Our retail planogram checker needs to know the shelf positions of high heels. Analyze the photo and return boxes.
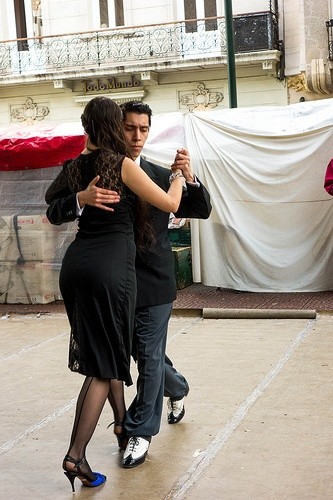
[113,421,126,448]
[63,454,106,492]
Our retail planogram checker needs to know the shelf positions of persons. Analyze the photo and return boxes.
[45,101,212,467]
[44,97,187,494]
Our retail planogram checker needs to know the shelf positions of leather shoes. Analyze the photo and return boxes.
[167,385,189,424]
[121,436,151,468]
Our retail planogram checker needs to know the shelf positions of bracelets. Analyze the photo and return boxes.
[169,174,184,183]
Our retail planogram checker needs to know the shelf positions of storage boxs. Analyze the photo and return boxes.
[167,218,193,290]
[0,217,78,304]
